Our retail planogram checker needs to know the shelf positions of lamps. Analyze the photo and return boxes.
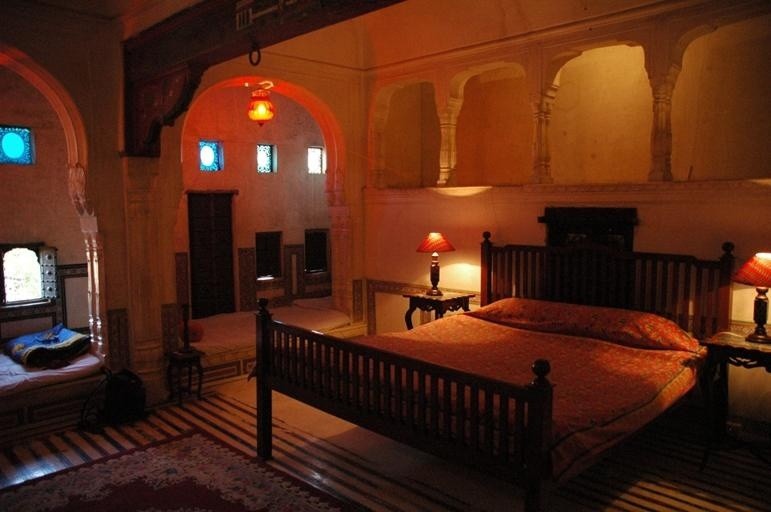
[248,80,278,128]
[730,251,771,345]
[416,231,459,296]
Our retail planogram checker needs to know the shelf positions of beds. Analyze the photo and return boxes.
[178,303,365,390]
[0,330,101,440]
[252,204,741,510]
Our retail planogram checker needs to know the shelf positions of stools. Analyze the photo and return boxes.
[165,348,204,410]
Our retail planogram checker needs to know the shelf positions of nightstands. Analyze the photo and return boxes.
[401,292,477,330]
[698,330,771,463]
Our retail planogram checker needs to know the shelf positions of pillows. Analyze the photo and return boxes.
[179,318,205,344]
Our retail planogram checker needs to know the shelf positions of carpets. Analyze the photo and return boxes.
[1,422,374,511]
